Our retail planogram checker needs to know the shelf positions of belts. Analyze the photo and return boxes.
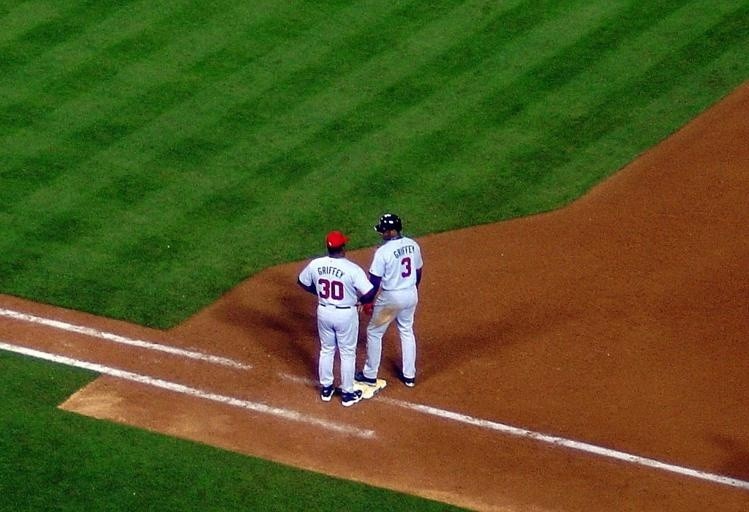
[321,304,351,309]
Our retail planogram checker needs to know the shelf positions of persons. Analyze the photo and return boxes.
[365,213,425,387]
[296,230,376,409]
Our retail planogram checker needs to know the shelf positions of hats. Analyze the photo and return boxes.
[374,213,402,235]
[326,232,348,248]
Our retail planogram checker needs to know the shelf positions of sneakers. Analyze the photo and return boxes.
[355,371,377,385]
[321,385,335,401]
[399,371,415,387]
[341,390,363,407]
